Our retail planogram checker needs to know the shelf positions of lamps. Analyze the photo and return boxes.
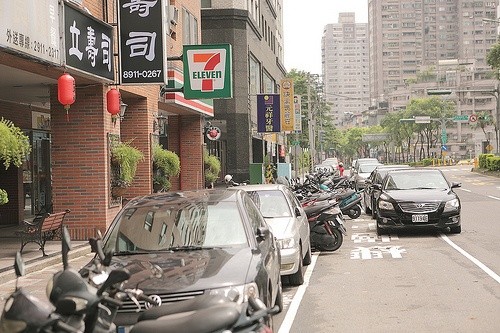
[151,113,166,136]
[119,99,128,122]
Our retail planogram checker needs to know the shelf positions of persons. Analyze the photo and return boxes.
[339,163,344,178]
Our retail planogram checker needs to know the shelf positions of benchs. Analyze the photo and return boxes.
[16,209,71,256]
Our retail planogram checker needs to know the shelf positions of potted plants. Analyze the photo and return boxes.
[204,154,222,186]
[150,143,181,194]
[106,131,145,198]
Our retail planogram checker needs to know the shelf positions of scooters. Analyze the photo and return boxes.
[0,226,280,333]
[285,167,363,251]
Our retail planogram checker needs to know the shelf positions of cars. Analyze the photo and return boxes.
[377,168,463,236]
[314,158,341,177]
[354,163,384,190]
[350,160,356,176]
[228,184,311,286]
[75,189,283,333]
[350,158,378,177]
[363,165,413,220]
